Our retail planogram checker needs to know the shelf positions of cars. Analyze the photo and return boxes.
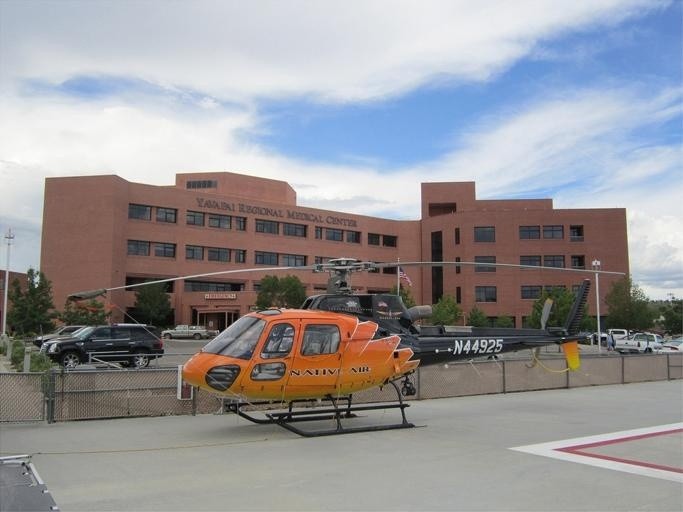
[575,328,682,354]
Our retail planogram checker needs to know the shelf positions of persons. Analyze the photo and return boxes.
[607,333,612,351]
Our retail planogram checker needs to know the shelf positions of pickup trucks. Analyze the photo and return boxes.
[161,324,210,340]
[189,325,220,338]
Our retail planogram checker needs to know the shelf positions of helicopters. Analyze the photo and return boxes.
[65,253,626,438]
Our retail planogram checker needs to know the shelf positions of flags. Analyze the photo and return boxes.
[398,266,414,288]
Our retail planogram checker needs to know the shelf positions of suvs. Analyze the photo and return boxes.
[33,322,165,369]
